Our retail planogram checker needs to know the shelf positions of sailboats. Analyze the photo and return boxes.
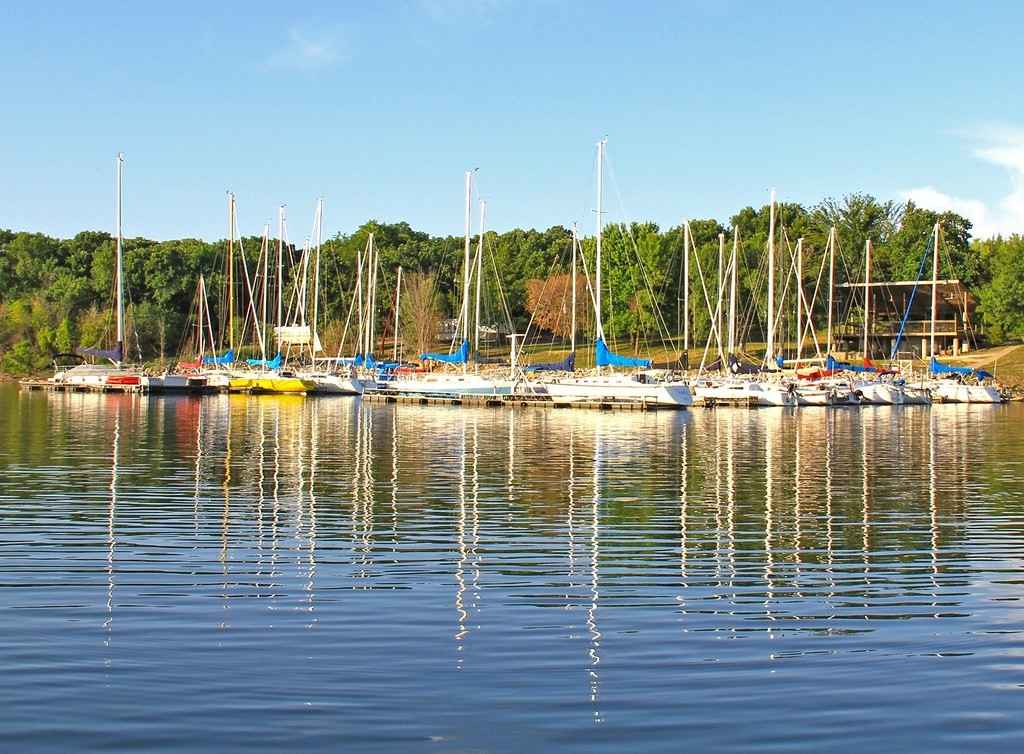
[51,135,1002,405]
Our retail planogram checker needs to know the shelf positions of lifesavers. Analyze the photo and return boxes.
[665,371,675,382]
